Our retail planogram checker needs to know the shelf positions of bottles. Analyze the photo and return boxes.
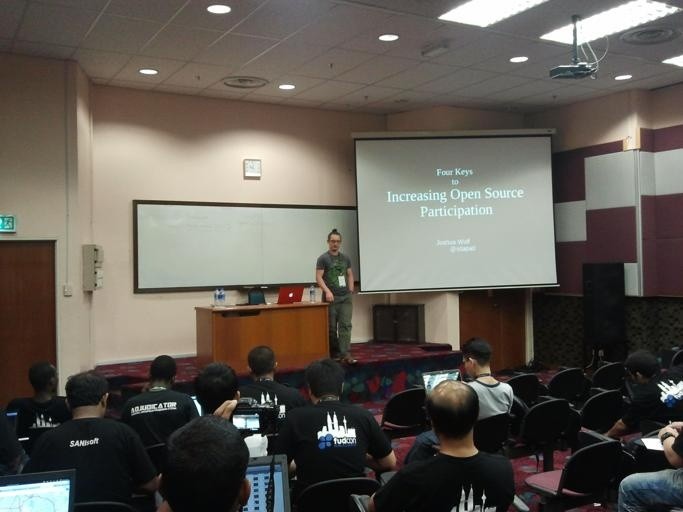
[309,285,316,304]
[214,288,225,307]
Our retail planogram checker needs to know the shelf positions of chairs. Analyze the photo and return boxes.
[248,288,266,305]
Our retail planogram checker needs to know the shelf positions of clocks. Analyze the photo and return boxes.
[244,159,262,178]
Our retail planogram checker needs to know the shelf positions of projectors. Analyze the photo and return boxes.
[549,61,596,80]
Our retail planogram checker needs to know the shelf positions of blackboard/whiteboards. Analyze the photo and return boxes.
[133,200,361,294]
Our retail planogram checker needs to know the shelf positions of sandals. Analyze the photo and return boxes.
[339,354,359,365]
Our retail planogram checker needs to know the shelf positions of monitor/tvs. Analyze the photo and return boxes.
[0,468,76,512]
[242,454,291,512]
[277,285,304,303]
[422,368,463,396]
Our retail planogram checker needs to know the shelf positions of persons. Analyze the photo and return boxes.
[316,232,359,366]
[1,338,683,512]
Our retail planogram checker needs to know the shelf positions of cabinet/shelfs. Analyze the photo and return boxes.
[372,303,425,343]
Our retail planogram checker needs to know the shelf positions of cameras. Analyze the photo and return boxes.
[229,397,279,436]
